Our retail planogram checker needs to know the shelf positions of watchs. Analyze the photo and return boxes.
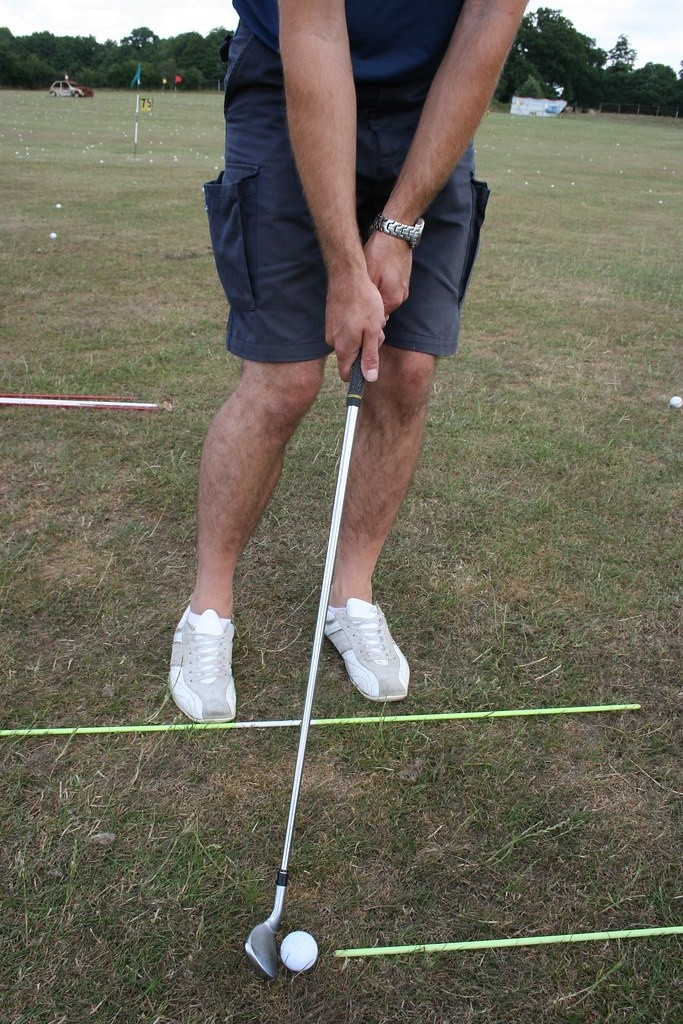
[372,214,425,247]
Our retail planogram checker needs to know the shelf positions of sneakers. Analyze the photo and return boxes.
[324,597,410,702]
[168,606,237,723]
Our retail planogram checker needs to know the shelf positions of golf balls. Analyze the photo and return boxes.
[56,204,61,208]
[670,396,683,409]
[279,928,319,974]
[49,233,57,239]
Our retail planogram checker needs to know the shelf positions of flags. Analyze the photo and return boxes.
[176,76,182,82]
[163,79,167,84]
[130,64,141,87]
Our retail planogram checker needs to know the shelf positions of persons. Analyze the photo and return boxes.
[166,0,528,724]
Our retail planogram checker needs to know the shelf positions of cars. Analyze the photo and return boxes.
[50,80,94,98]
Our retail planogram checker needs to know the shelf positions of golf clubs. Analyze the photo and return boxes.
[242,355,366,984]
